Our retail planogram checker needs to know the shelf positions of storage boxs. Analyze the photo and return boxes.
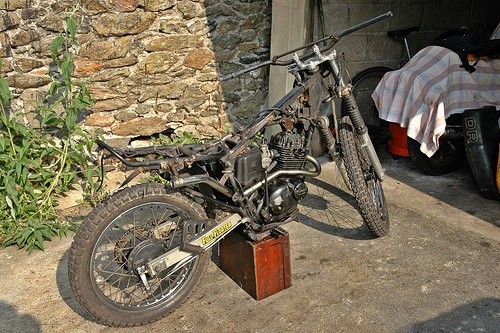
[217,227,291,301]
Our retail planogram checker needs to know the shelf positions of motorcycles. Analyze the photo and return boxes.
[68,11,394,328]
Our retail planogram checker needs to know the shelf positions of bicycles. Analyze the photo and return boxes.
[340,26,419,146]
